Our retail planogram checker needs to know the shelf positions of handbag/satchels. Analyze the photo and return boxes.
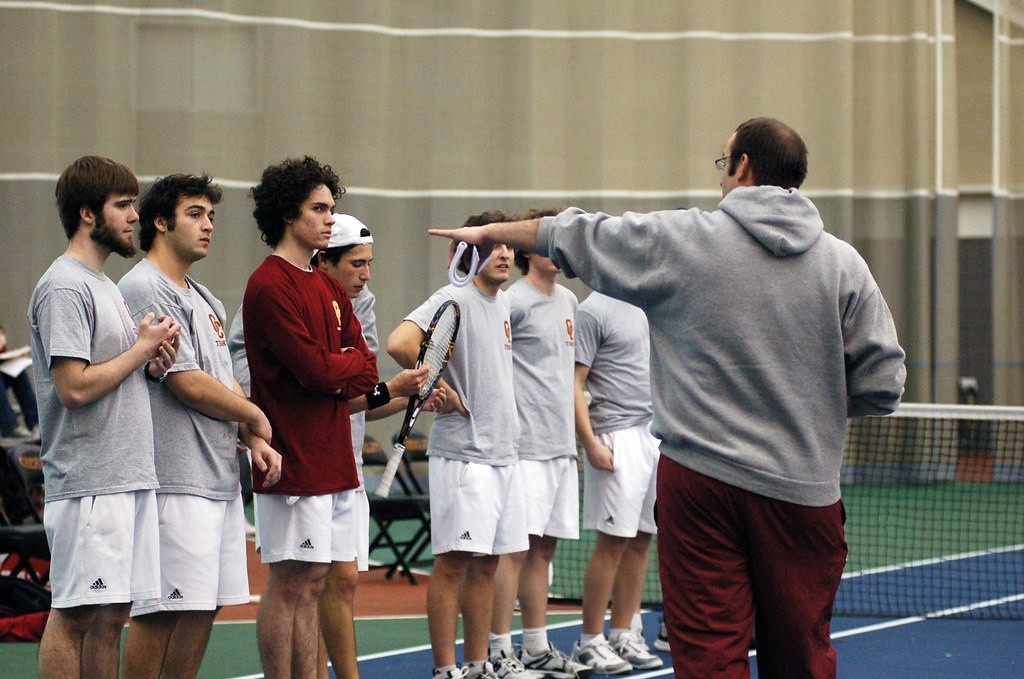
[0,544,53,644]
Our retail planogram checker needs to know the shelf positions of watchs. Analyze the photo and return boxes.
[144,361,169,383]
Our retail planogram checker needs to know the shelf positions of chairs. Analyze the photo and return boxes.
[394,428,443,577]
[355,435,431,586]
[0,445,53,589]
[6,442,48,522]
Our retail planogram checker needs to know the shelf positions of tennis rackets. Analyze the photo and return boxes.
[374,300,462,500]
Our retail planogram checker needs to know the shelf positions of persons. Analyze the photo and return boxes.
[228,212,447,678]
[28,153,182,679]
[384,209,531,679]
[433,115,906,679]
[115,168,283,679]
[504,204,595,679]
[242,155,379,677]
[573,283,664,676]
[654,614,670,652]
[0,326,39,531]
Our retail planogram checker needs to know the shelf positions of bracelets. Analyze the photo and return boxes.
[365,382,390,410]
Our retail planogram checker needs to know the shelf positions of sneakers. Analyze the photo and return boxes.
[432,665,469,679]
[488,645,548,679]
[464,662,499,679]
[518,643,594,679]
[604,632,662,670]
[571,637,633,674]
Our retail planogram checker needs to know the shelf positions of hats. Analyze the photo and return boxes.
[312,212,372,259]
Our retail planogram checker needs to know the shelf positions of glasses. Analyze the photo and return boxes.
[715,156,746,174]
